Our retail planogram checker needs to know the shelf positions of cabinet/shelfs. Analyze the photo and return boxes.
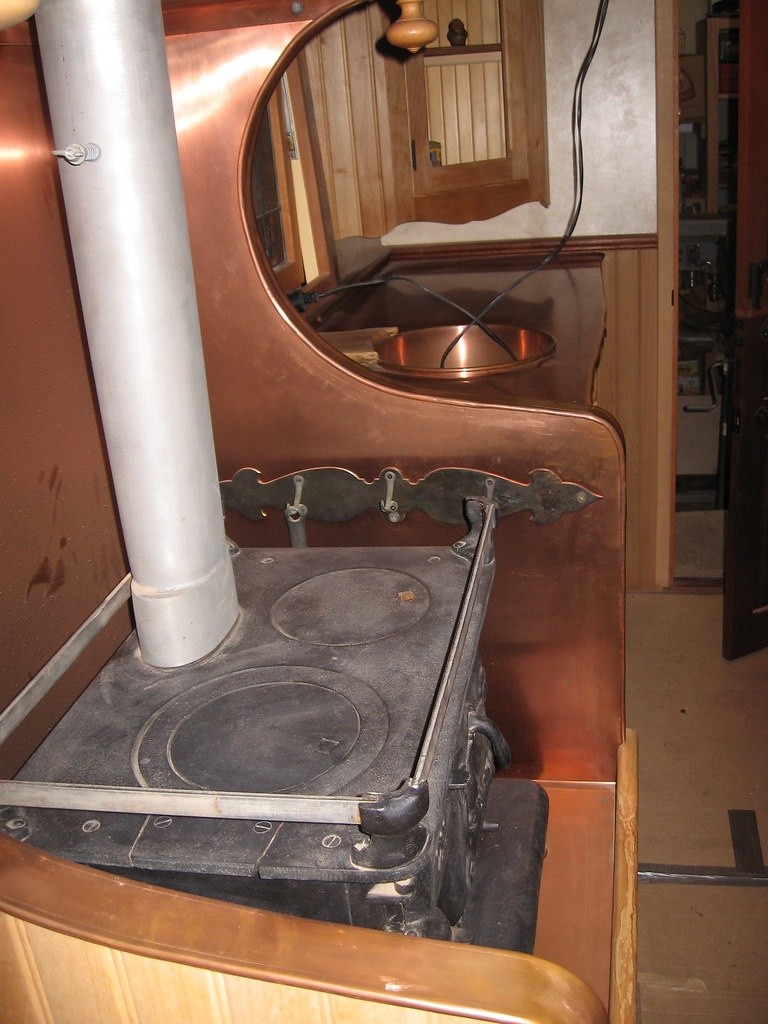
[304,0,551,240]
[678,53,705,123]
[706,16,738,214]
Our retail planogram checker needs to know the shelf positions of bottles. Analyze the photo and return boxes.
[687,244,722,303]
[719,29,731,61]
[679,159,699,203]
[678,23,690,56]
